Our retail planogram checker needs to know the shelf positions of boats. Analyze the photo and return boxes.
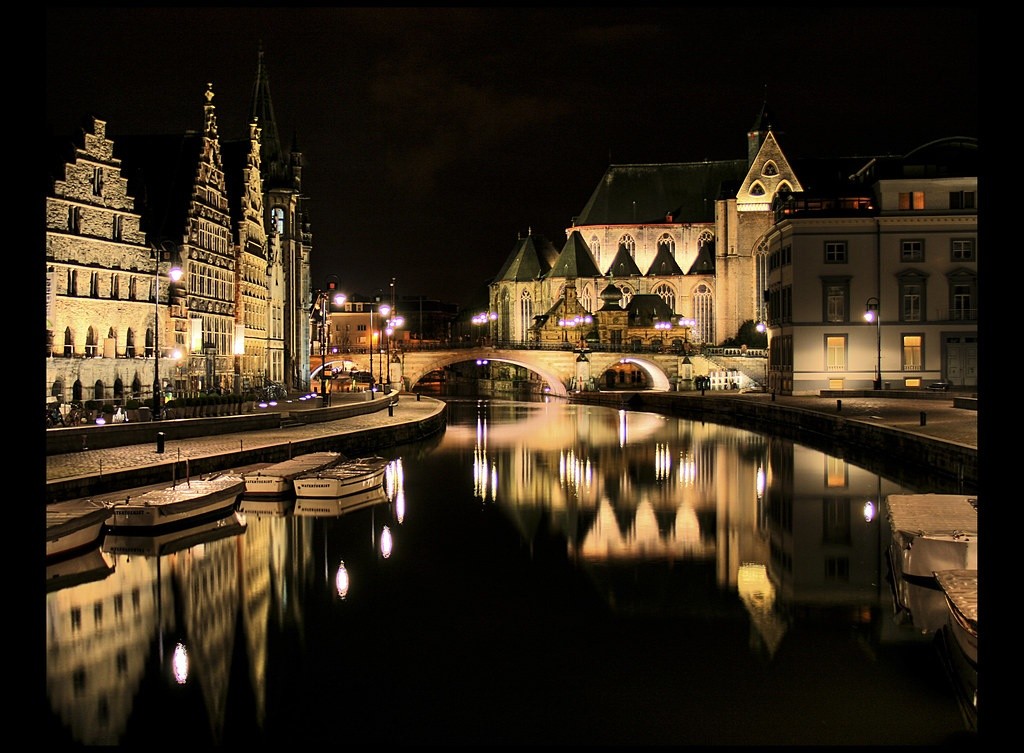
[46,547,117,590]
[99,508,248,558]
[103,459,248,533]
[295,457,391,498]
[47,509,104,565]
[244,452,344,497]
[236,499,293,518]
[289,485,389,519]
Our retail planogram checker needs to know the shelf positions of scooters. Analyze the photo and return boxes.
[47,400,66,428]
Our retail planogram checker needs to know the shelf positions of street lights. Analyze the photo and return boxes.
[866,296,883,390]
[679,320,696,355]
[371,299,393,400]
[320,293,347,406]
[756,320,784,395]
[472,312,488,349]
[655,322,671,345]
[573,316,594,353]
[153,240,184,422]
[378,309,406,391]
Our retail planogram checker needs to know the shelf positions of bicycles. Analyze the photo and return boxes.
[63,402,84,427]
[254,377,287,403]
